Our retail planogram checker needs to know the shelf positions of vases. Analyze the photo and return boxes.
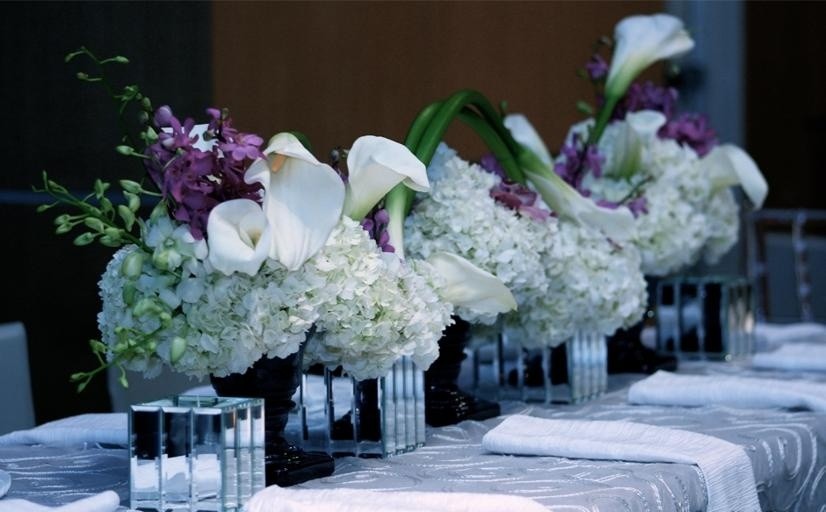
[332,314,502,445]
[207,319,335,487]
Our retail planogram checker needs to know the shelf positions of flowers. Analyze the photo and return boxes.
[27,13,773,402]
